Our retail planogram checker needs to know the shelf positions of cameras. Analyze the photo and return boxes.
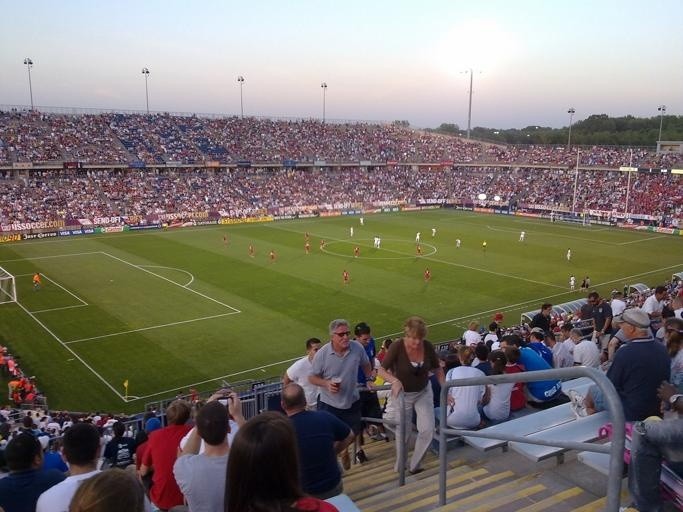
[217,397,233,406]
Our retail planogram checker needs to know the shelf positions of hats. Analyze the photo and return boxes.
[527,327,544,338]
[496,313,503,321]
[613,308,650,330]
[145,418,161,432]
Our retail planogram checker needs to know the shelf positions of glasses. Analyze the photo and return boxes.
[334,331,350,337]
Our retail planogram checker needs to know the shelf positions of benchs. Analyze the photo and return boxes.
[317,316,683,512]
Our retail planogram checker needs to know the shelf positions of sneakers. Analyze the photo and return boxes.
[355,450,366,462]
[340,448,351,470]
[567,390,588,419]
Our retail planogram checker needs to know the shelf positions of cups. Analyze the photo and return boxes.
[330,376,342,392]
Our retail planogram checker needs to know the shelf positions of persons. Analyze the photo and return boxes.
[304,231,325,253]
[373,236,380,248]
[424,267,431,280]
[354,247,360,256]
[282,318,387,471]
[33,272,41,289]
[520,231,525,242]
[569,275,591,293]
[375,272,682,511]
[282,383,355,502]
[483,240,489,250]
[415,228,437,254]
[223,414,341,511]
[456,238,461,249]
[0,347,249,512]
[0,106,682,240]
[567,248,571,261]
[350,217,364,236]
[341,270,350,284]
[223,237,275,259]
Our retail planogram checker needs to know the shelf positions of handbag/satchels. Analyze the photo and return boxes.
[599,422,632,464]
